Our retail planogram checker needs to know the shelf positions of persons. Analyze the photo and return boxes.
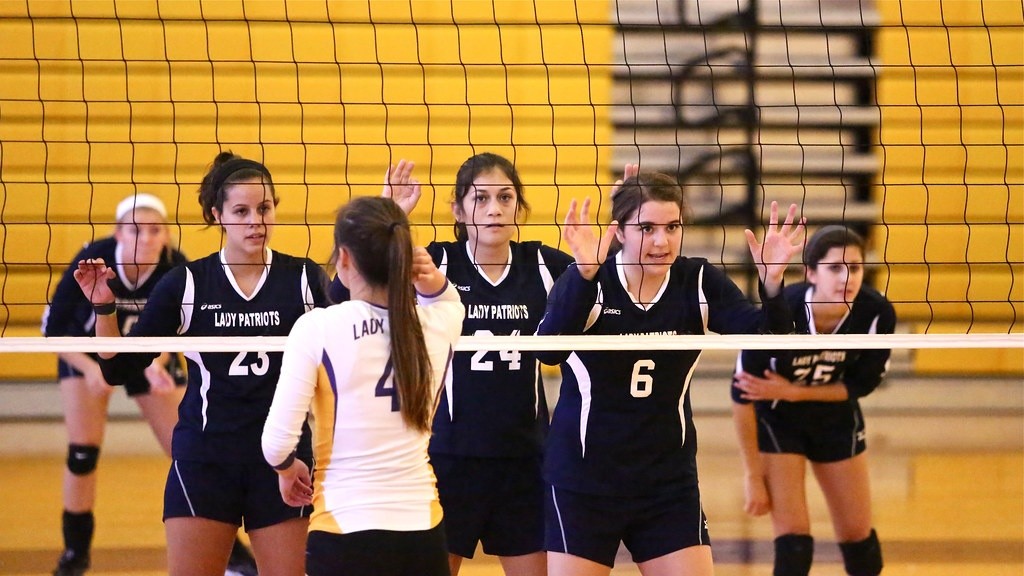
[533,173,808,576]
[325,151,640,576]
[261,196,466,576]
[730,227,897,576]
[73,152,331,576]
[40,195,189,576]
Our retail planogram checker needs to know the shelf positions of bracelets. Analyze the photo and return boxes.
[92,301,115,316]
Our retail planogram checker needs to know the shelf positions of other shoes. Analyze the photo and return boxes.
[51,548,91,576]
[225,538,258,576]
